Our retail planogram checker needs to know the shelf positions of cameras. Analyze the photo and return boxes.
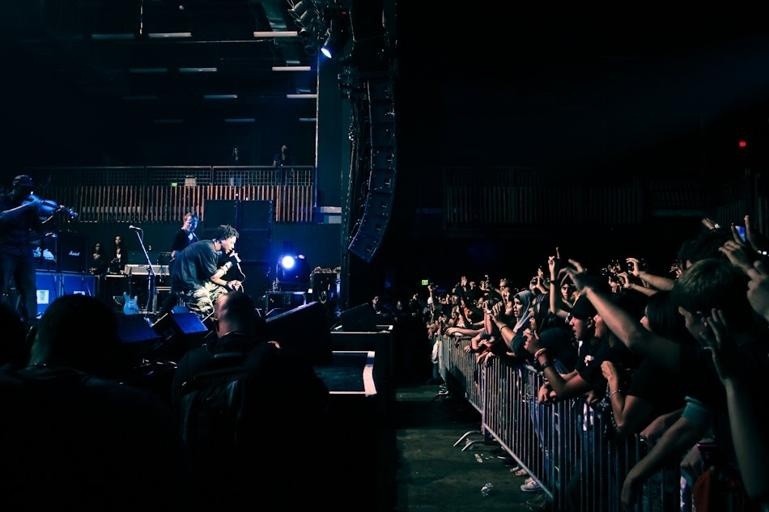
[628,258,649,271]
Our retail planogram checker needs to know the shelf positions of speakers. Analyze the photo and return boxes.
[32,268,156,316]
[113,301,370,367]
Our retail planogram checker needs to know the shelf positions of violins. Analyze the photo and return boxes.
[22,194,78,217]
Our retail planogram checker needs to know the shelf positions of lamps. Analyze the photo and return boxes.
[227,141,291,169]
[319,16,343,59]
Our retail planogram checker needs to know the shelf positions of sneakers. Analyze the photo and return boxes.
[485,432,542,494]
[438,382,449,397]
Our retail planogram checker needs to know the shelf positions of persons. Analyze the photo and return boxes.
[231,145,241,165]
[272,144,289,170]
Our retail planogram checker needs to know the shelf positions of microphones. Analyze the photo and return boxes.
[128,225,141,233]
[230,250,243,263]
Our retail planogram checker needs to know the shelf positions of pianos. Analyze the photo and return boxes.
[125,264,170,276]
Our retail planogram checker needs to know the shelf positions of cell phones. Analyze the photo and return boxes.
[732,224,746,243]
